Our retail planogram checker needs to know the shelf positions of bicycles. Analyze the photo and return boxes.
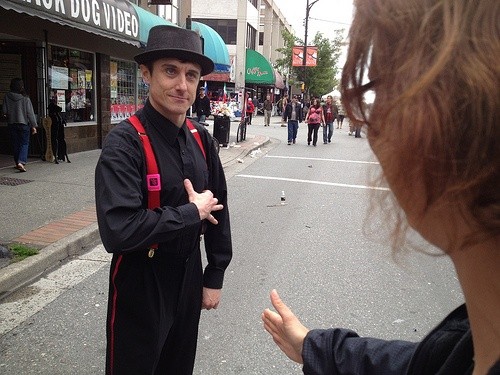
[237,116,249,142]
[186,116,220,154]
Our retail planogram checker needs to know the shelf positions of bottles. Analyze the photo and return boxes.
[281,191,286,206]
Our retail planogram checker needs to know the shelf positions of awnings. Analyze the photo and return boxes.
[191,20,231,72]
[246,49,285,89]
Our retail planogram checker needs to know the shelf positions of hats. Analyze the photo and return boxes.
[10,77,24,90]
[200,87,205,90]
[129,24,214,78]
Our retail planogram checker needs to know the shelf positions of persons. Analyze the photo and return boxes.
[1,77,37,172]
[246,95,259,125]
[348,119,361,138]
[281,93,346,145]
[262,0,500,375]
[95,24,232,375]
[192,87,212,127]
[262,96,273,126]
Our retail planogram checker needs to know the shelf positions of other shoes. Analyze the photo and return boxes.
[293,140,295,144]
[308,142,310,145]
[17,163,27,173]
[328,140,331,142]
[324,141,327,144]
[313,143,316,146]
[288,142,292,145]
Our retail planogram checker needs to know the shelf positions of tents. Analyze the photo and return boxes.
[321,89,342,99]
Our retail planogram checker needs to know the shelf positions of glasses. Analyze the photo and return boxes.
[341,76,384,125]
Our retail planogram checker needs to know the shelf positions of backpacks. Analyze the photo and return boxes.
[247,105,251,111]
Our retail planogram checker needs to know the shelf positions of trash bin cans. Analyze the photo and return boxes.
[212,115,231,147]
